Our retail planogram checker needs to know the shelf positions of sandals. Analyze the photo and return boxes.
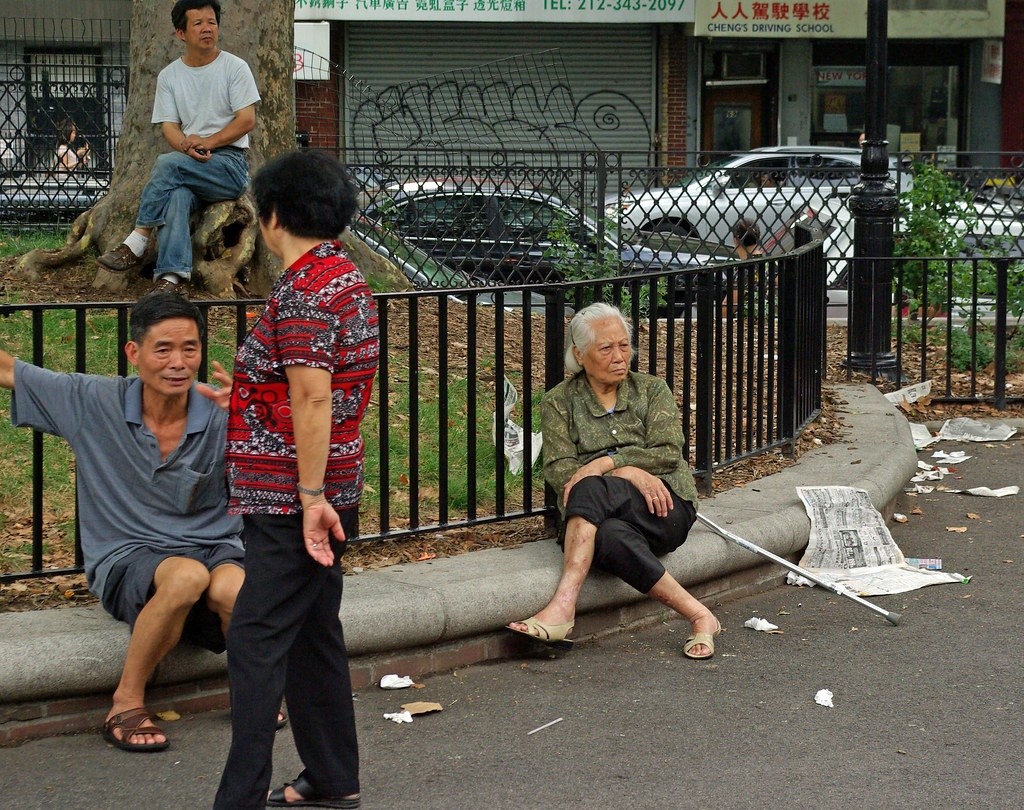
[505,611,575,649]
[683,617,721,661]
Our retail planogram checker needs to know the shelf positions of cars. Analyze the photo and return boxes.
[356,180,743,319]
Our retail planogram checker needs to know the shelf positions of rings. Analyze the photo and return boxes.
[313,544,317,546]
[653,497,658,500]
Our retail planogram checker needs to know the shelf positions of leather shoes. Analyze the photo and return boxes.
[96,243,149,273]
[148,277,188,299]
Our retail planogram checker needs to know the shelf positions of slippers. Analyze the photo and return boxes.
[277,705,288,727]
[267,770,362,808]
[101,703,171,753]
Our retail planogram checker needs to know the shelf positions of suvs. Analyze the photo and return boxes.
[592,146,916,247]
[757,191,1024,323]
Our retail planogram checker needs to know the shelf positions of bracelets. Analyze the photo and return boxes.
[297,482,325,496]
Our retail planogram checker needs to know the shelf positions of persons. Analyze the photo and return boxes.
[53,123,87,181]
[0,292,288,752]
[197,150,379,810]
[720,218,779,317]
[506,301,721,660]
[96,0,260,297]
